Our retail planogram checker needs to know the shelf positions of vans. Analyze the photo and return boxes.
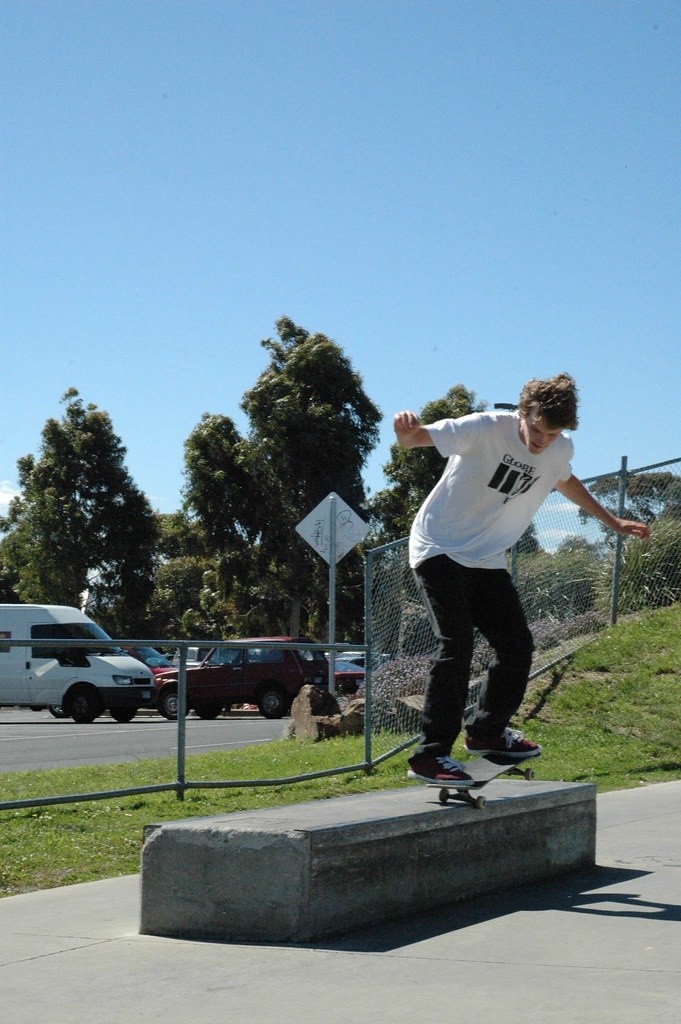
[0,603,156,726]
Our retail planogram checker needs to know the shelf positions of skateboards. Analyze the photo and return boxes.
[427,744,543,809]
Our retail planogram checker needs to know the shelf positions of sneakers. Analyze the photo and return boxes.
[406,757,474,786]
[463,726,542,758]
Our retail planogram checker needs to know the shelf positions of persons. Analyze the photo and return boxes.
[394,373,652,788]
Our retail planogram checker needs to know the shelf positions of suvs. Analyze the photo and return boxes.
[153,635,329,721]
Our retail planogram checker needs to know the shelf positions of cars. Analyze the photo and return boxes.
[123,644,391,695]
